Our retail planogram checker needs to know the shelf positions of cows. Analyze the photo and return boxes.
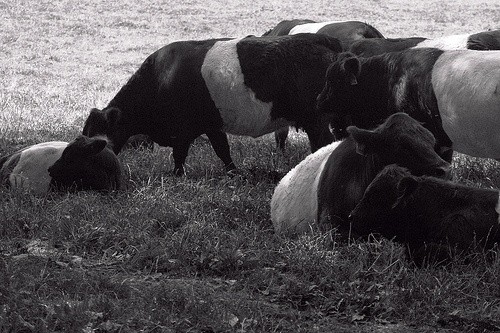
[315,47,500,163]
[347,36,500,58]
[261,19,385,155]
[270,113,452,238]
[82,33,342,178]
[0,134,128,196]
[347,164,500,268]
